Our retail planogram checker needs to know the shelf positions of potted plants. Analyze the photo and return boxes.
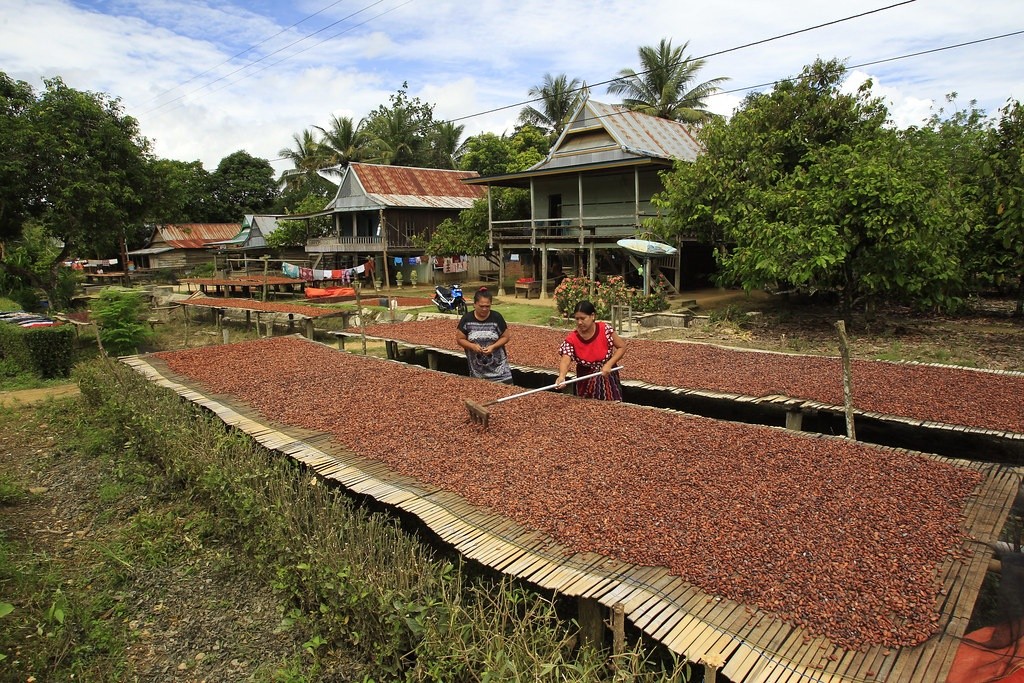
[375,277,383,291]
[351,279,359,295]
[395,272,404,290]
[96,264,103,274]
[410,270,418,289]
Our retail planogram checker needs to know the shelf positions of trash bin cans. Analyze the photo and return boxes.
[127,261,134,271]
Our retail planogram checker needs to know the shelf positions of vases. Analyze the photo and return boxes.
[626,290,633,297]
[636,290,643,297]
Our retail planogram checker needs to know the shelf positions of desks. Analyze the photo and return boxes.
[515,281,541,300]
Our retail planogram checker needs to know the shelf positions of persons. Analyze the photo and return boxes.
[97,266,103,274]
[455,286,513,386]
[554,301,627,402]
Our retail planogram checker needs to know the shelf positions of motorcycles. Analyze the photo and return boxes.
[431,280,468,316]
